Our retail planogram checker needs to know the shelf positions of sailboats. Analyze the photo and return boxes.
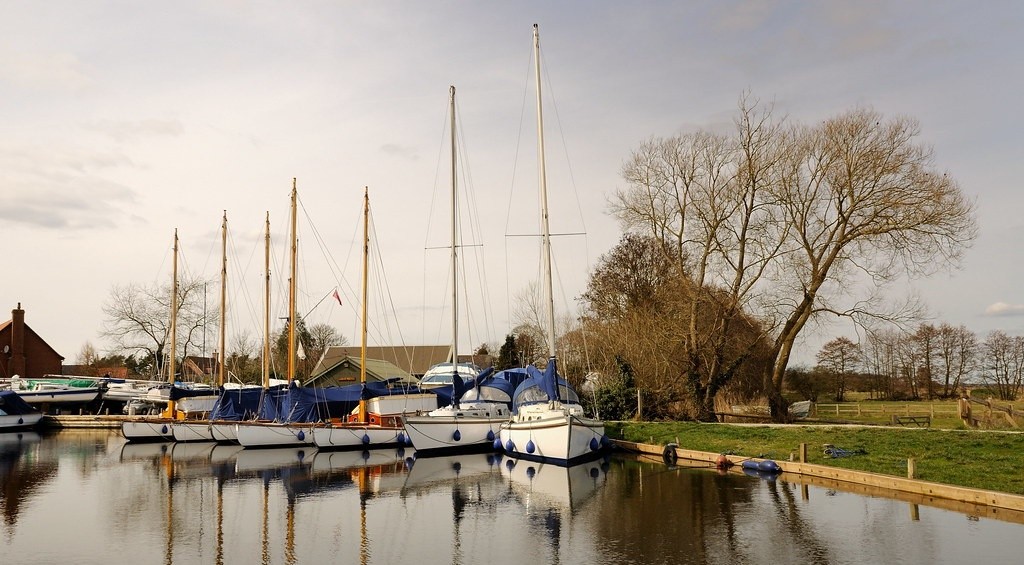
[401,86,529,454]
[119,440,416,565]
[492,452,610,565]
[122,178,485,448]
[400,449,499,565]
[501,23,605,464]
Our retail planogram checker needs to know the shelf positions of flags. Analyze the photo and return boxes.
[333,291,341,305]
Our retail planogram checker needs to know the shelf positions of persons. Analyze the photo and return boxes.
[453,371,465,407]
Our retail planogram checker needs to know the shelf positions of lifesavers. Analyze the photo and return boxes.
[661,444,679,469]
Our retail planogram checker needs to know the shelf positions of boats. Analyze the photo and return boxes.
[0,375,208,429]
